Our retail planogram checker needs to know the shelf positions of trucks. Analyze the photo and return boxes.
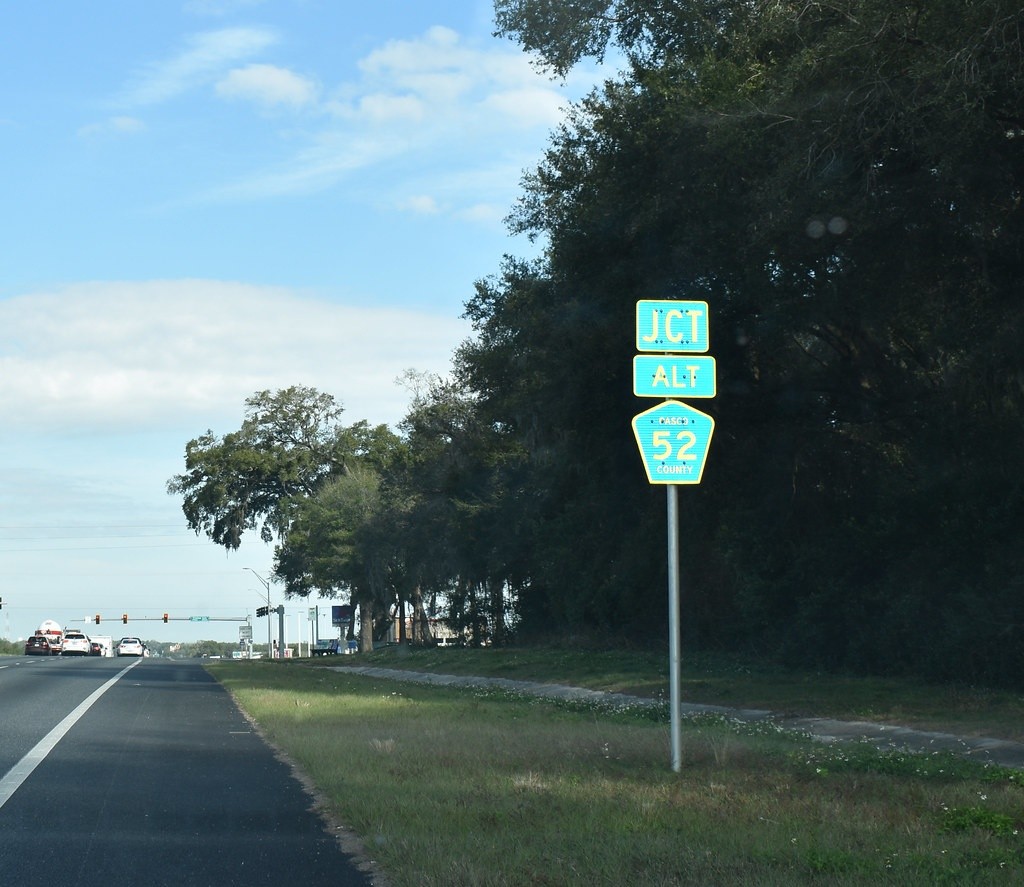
[34,620,80,656]
[88,635,114,658]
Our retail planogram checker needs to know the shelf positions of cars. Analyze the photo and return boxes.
[25,637,51,656]
[121,637,151,658]
[61,633,91,656]
[90,643,104,657]
[117,639,142,657]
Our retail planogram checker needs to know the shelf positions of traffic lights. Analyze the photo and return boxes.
[164,614,168,623]
[95,615,100,624]
[256,607,268,618]
[122,615,128,624]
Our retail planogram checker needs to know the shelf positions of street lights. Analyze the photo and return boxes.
[242,568,271,659]
